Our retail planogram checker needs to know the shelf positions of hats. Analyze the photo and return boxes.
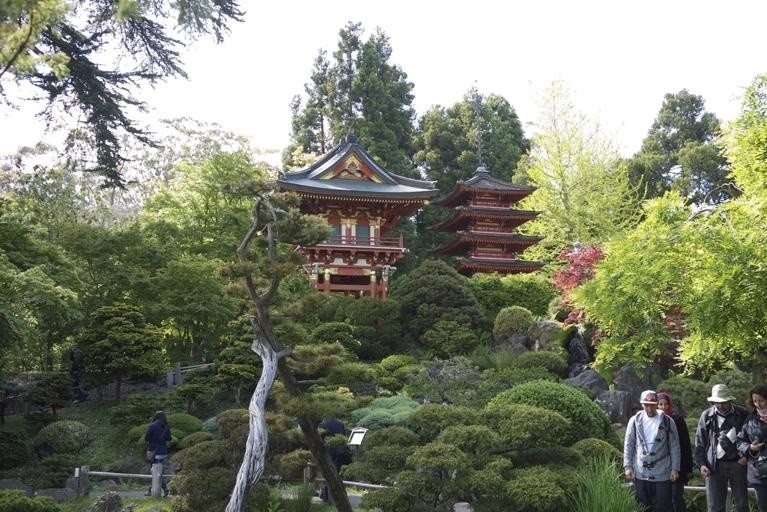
[639,389,658,405]
[706,384,736,402]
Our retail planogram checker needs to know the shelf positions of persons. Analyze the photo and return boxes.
[738,385,767,512]
[143,408,173,497]
[620,389,680,511]
[317,419,353,502]
[655,391,694,512]
[693,383,751,512]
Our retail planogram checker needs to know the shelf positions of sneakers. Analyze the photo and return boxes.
[162,487,168,497]
[143,491,151,495]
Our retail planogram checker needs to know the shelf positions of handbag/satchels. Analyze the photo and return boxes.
[145,450,156,464]
[334,445,352,464]
[753,458,766,480]
